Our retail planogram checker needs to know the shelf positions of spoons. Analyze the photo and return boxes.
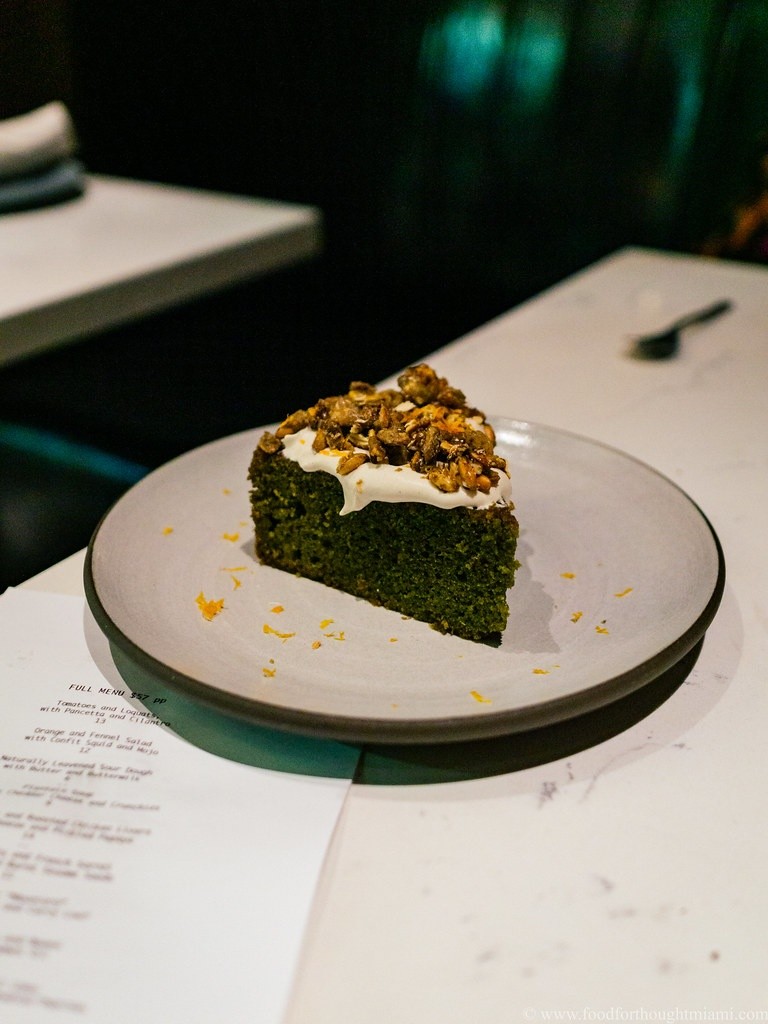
[633,300,730,356]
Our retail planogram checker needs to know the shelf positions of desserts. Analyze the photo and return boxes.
[246,364,521,646]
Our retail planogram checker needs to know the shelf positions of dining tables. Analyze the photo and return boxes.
[1,165,321,363]
[0,244,768,1024]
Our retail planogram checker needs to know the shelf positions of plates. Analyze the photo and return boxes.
[84,416,725,744]
